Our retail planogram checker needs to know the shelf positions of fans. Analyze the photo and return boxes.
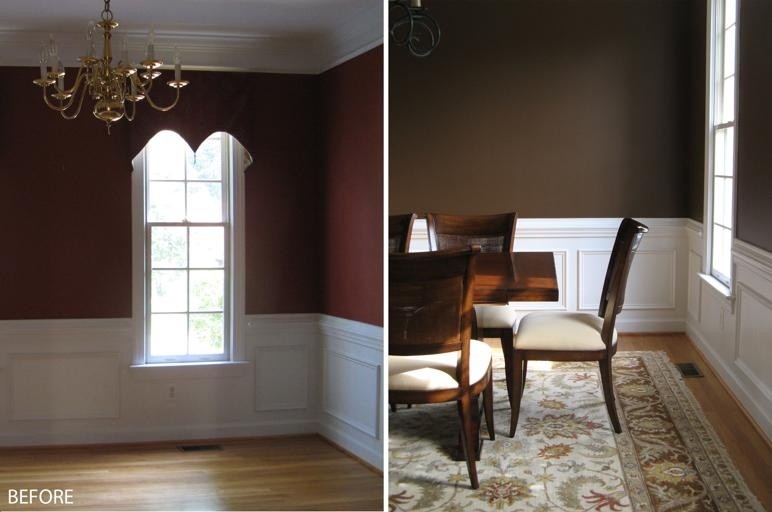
[388,249,559,460]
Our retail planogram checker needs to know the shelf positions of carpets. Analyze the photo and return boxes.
[389,348,768,511]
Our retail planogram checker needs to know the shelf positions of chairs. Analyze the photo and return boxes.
[387,245,498,487]
[388,212,419,252]
[425,204,519,402]
[511,217,649,438]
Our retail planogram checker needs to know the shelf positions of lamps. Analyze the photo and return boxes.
[33,0,190,136]
[390,0,441,58]
